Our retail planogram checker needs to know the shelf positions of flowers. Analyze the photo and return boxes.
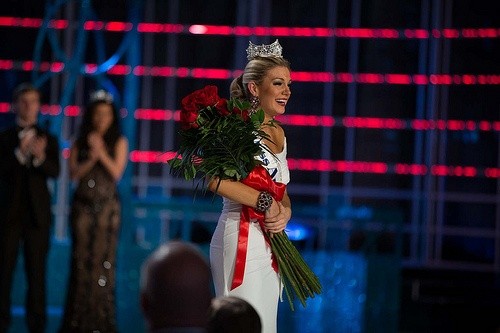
[159,86,321,312]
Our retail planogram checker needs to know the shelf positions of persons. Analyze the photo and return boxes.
[138,241,262,333]
[0,82,61,333]
[209,39,292,333]
[61,89,128,332]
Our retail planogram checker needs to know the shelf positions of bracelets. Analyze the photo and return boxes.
[256,191,273,212]
